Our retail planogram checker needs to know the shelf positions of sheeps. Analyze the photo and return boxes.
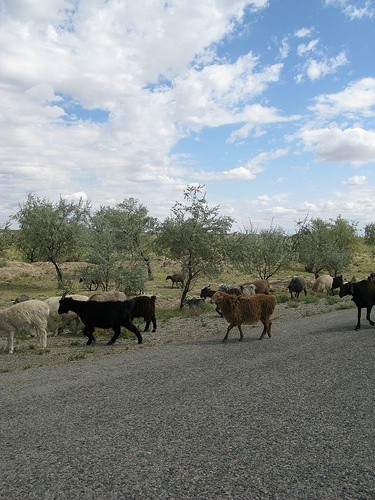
[200,281,271,318]
[14,294,30,306]
[338,277,375,330]
[210,292,277,342]
[0,300,49,353]
[164,274,187,289]
[87,290,127,303]
[130,296,156,334]
[59,289,144,346]
[314,273,357,296]
[288,277,308,298]
[45,295,89,337]
